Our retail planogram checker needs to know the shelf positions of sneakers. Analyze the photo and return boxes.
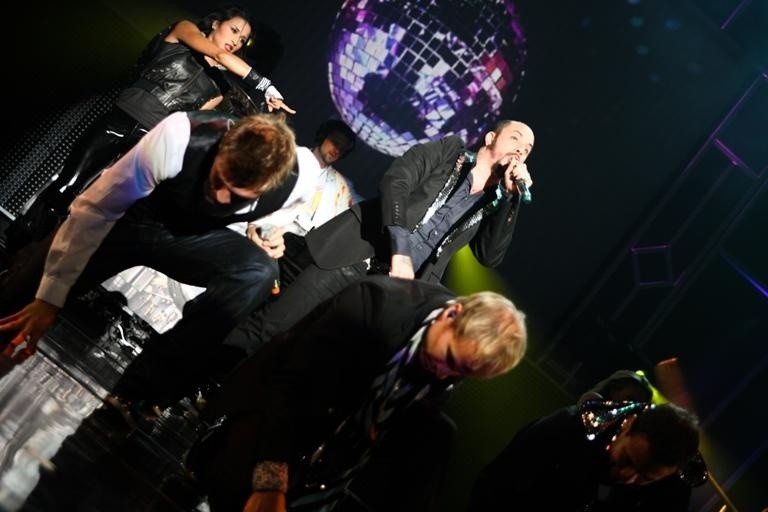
[102,392,142,431]
[184,422,230,472]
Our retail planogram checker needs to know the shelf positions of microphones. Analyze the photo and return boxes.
[512,165,532,205]
[259,222,281,295]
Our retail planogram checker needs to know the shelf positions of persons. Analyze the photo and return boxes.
[3,2,536,510]
[461,396,709,510]
[568,367,653,415]
